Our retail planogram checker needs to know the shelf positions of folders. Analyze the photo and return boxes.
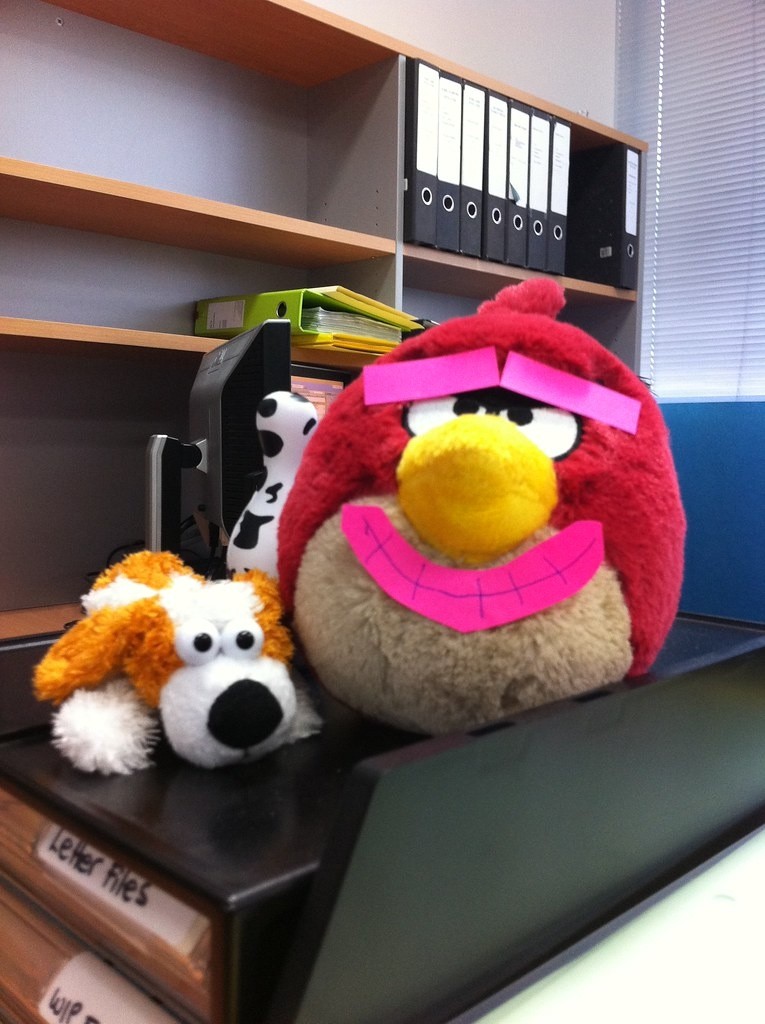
[403,57,441,247]
[459,80,488,259]
[544,117,572,276]
[525,108,552,272]
[503,99,532,268]
[565,144,641,290]
[431,72,463,253]
[192,288,411,340]
[480,88,510,264]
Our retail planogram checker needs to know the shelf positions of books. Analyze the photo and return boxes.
[290,375,344,424]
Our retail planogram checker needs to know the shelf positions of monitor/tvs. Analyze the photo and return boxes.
[143,315,292,557]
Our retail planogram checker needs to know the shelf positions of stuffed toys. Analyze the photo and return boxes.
[223,392,319,580]
[273,276,684,743]
[29,549,300,779]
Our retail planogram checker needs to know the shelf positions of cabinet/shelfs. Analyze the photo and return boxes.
[0,0,646,649]
